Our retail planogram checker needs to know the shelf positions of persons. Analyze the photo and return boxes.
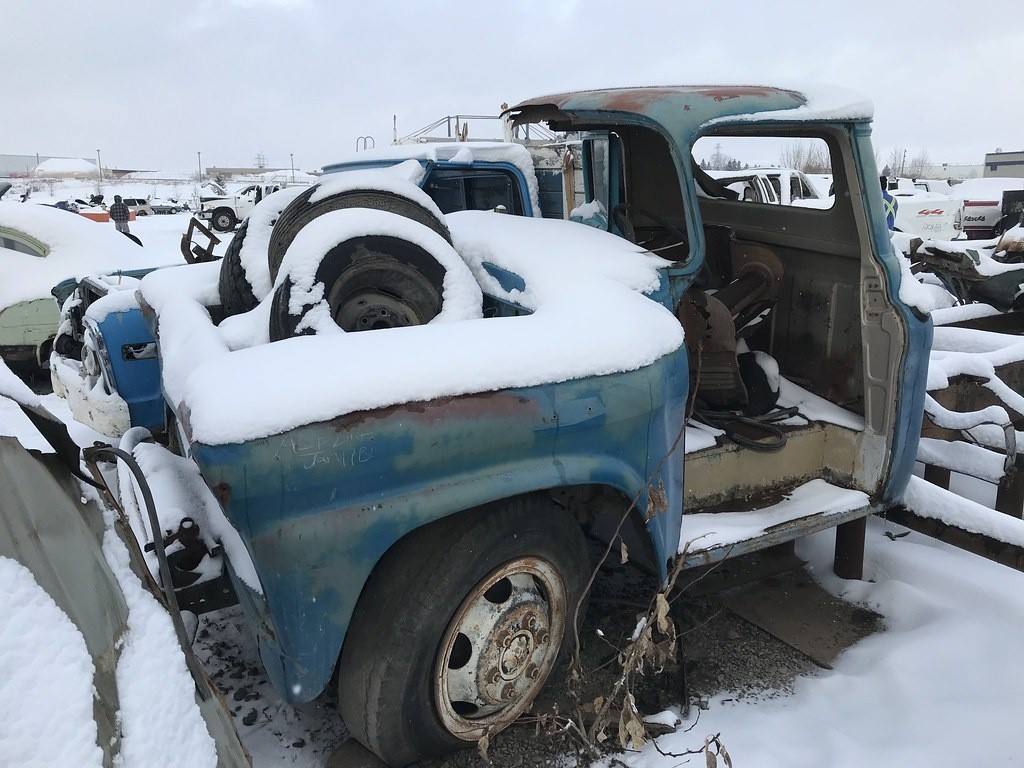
[879,176,898,231]
[110,195,130,233]
